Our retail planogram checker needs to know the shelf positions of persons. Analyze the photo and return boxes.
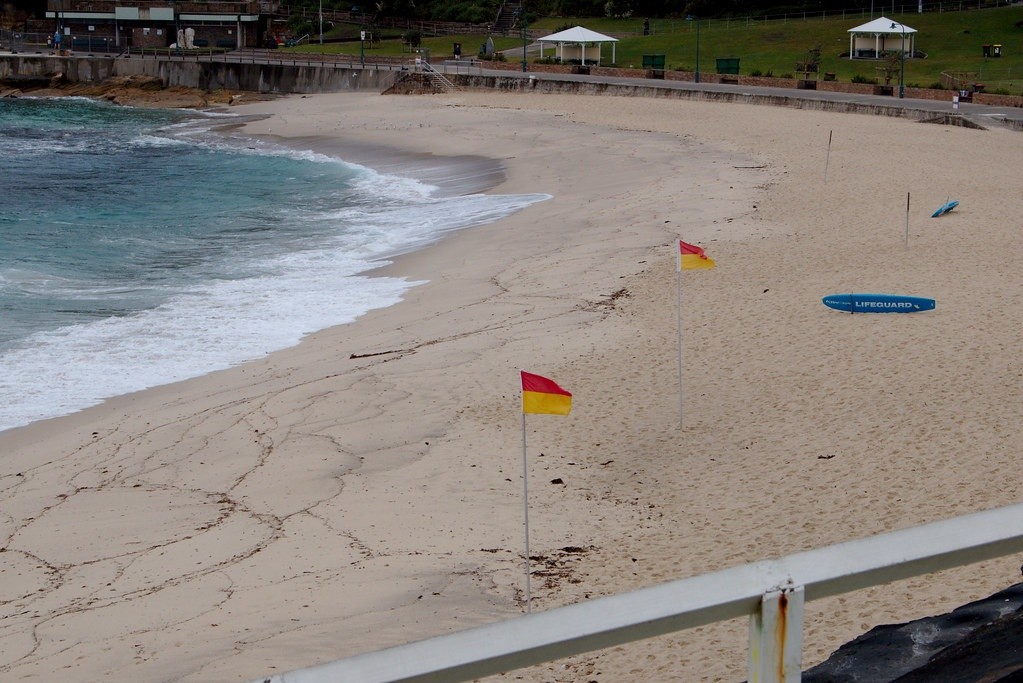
[54,32,60,49]
[480,42,487,54]
[644,18,649,35]
[455,45,461,59]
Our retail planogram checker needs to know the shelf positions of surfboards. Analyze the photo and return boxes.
[821,294,935,314]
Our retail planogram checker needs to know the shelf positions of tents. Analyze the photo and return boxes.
[847,17,918,60]
[537,26,620,66]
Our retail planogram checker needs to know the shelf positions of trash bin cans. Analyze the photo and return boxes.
[453,42,462,61]
[415,48,430,73]
[982,44,991,58]
[46,35,52,48]
[994,45,1002,58]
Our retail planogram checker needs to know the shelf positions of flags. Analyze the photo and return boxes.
[521,370,572,416]
[677,239,715,272]
